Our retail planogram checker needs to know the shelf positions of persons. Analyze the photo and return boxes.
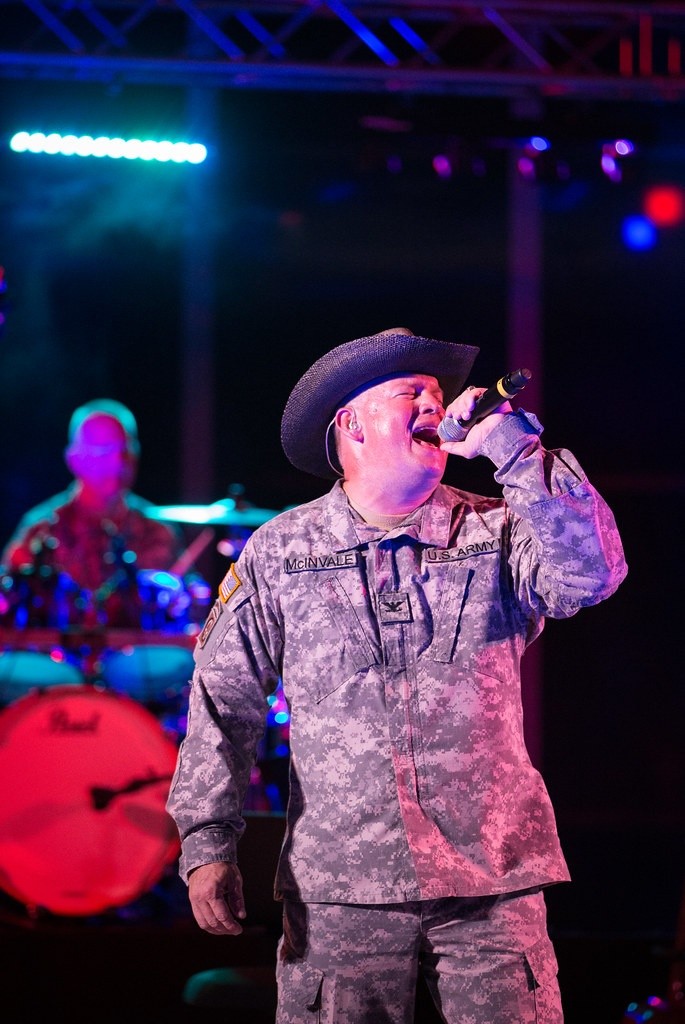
[1,396,184,626]
[165,326,627,1023]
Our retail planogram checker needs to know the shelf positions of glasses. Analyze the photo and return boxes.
[76,440,142,458]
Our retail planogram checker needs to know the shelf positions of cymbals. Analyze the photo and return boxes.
[141,497,283,527]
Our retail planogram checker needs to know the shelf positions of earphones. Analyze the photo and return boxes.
[348,420,356,430]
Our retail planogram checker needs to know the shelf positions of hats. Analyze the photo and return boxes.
[280,327,480,481]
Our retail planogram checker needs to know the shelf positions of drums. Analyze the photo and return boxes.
[0,682,181,925]
[0,561,211,709]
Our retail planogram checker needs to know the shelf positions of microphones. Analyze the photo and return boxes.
[437,368,532,443]
